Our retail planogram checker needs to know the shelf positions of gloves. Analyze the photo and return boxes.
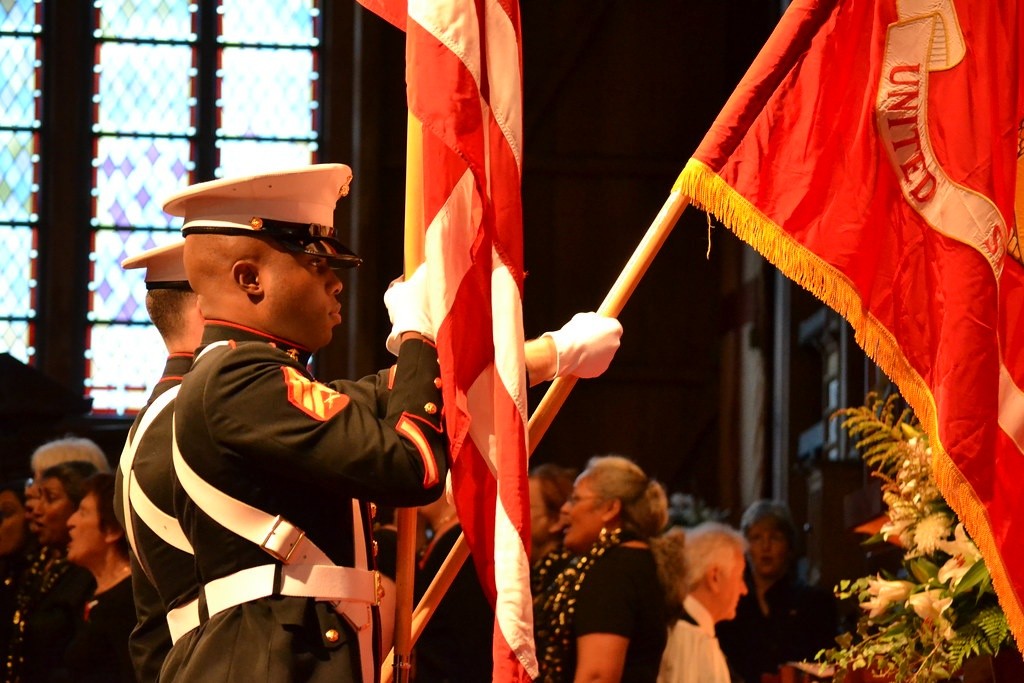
[539,312,623,381]
[384,263,436,357]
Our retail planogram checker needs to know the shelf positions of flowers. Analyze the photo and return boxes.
[797,391,1019,683]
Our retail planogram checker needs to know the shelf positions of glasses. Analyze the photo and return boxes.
[570,495,604,505]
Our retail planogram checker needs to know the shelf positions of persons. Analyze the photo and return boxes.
[528,455,819,683]
[116,163,623,683]
[0,437,133,683]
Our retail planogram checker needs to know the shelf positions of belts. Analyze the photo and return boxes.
[166,597,200,646]
[205,564,385,619]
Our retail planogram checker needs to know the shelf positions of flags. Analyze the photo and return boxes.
[356,0,538,683]
[672,0,1024,650]
